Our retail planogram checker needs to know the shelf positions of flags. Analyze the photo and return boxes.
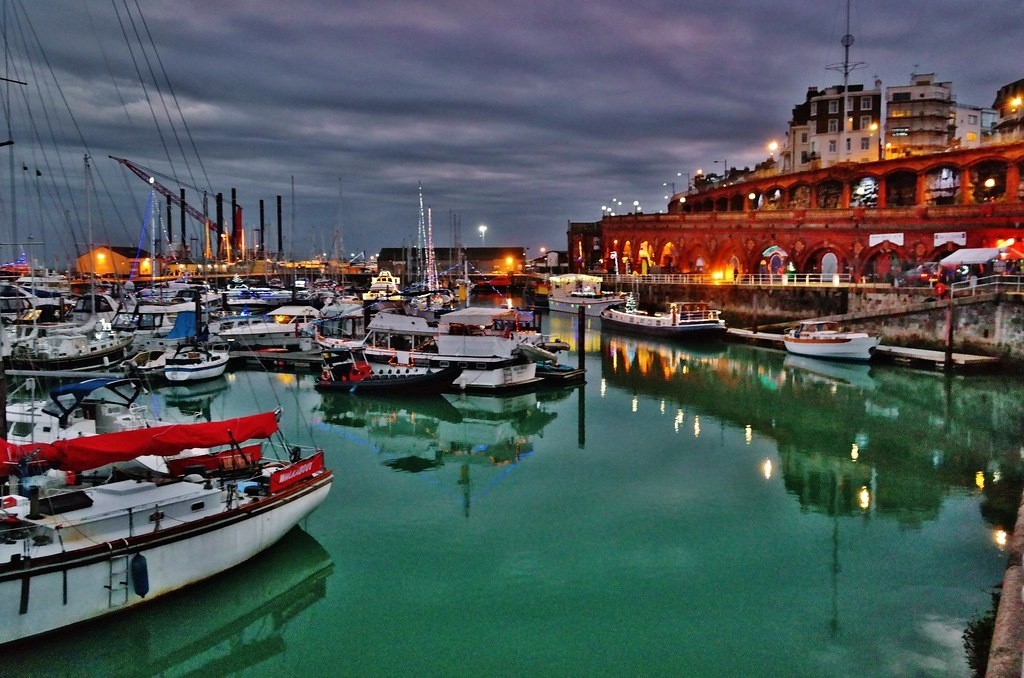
[0,255,28,272]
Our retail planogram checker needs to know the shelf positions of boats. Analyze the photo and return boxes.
[0,0,588,650]
[599,290,729,344]
[782,320,885,361]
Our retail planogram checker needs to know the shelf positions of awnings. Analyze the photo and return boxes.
[941,248,999,264]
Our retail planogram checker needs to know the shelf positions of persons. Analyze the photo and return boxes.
[1004,258,1022,292]
[957,261,969,288]
[939,266,946,283]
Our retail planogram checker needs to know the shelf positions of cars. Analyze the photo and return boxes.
[890,268,937,287]
